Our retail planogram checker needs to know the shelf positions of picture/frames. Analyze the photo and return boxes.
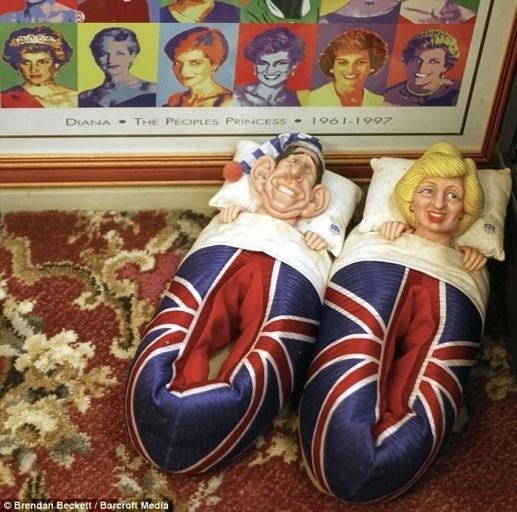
[0,0,517,187]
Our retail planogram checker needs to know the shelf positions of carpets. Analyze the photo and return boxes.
[1,212,515,510]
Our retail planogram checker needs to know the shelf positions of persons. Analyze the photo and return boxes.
[0,0,479,25]
[391,29,462,106]
[310,27,388,107]
[78,26,156,107]
[216,128,331,254]
[0,26,76,107]
[375,139,492,274]
[160,26,233,107]
[236,26,306,107]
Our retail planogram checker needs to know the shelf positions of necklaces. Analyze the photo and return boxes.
[25,81,54,87]
[406,80,442,97]
